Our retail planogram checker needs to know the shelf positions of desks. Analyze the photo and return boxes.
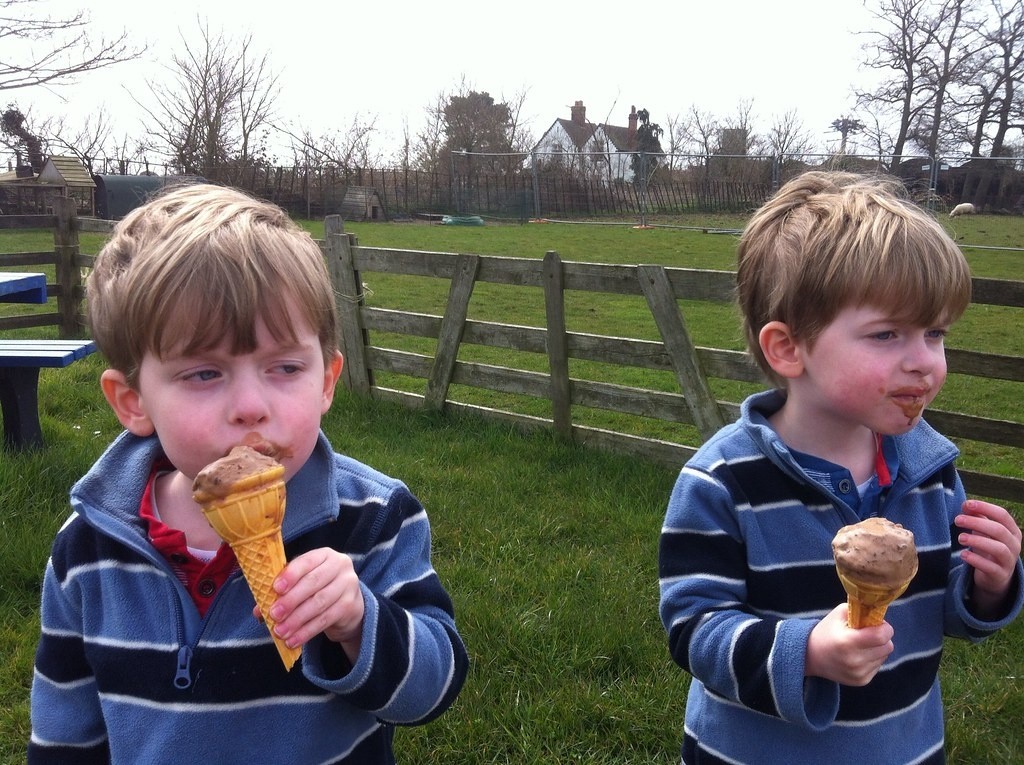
[1,271,47,305]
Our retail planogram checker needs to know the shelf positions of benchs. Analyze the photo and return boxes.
[0,339,98,453]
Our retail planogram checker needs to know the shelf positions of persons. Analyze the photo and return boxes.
[28,182,471,765]
[660,172,1024,765]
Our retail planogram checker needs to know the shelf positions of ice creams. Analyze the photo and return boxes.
[832,517,918,628]
[191,446,302,672]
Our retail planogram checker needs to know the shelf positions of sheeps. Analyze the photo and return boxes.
[949,202,975,220]
